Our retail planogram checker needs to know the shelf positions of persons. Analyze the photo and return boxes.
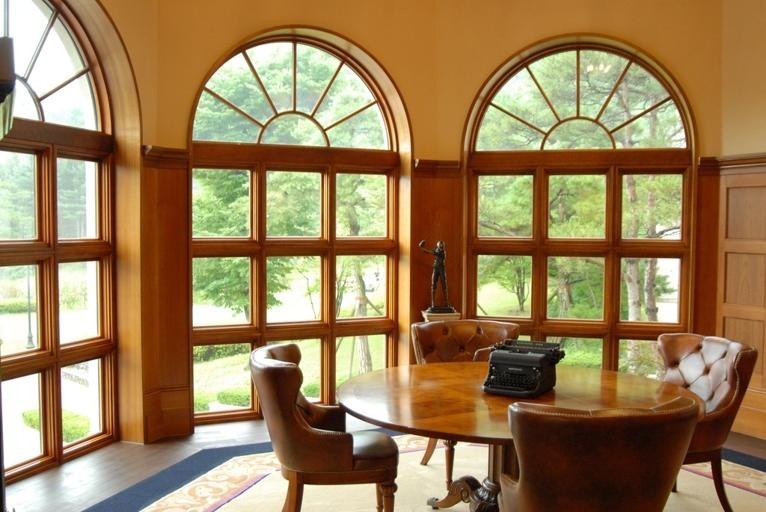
[417,240,450,308]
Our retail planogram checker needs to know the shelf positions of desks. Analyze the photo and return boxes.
[335,358,707,512]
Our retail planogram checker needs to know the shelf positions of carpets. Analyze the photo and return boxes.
[82,426,766,511]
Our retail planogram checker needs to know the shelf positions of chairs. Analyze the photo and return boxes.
[493,395,699,511]
[248,339,400,512]
[409,316,521,492]
[655,326,759,512]
